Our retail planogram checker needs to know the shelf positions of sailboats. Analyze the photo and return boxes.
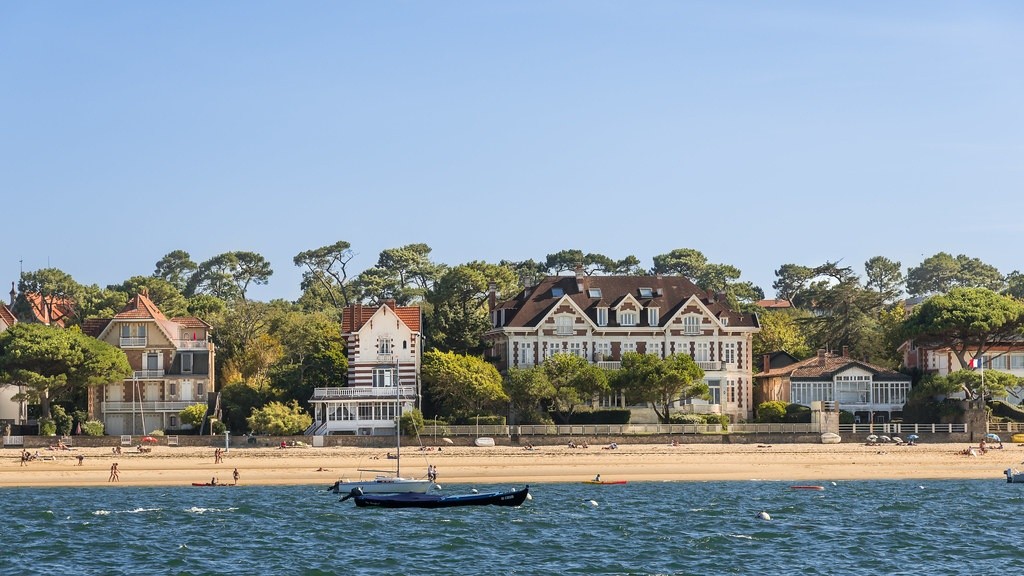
[336,359,434,494]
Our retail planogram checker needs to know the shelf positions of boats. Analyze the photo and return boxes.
[1004,469,1024,483]
[192,483,235,486]
[582,480,627,485]
[351,485,528,509]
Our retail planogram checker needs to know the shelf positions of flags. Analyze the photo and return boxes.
[970,358,981,367]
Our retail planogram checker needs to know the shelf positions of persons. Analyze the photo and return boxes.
[233,468,240,484]
[20,448,38,466]
[214,448,224,464]
[108,463,120,482]
[980,439,987,455]
[57,439,67,450]
[211,477,215,484]
[595,474,600,481]
[76,455,83,465]
[281,441,286,447]
[428,465,437,483]
[965,446,975,455]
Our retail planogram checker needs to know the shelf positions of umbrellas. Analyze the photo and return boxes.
[75,422,81,436]
[880,435,891,441]
[867,435,878,440]
[892,437,903,443]
[194,331,197,341]
[141,437,158,446]
[985,434,1000,441]
[907,434,919,439]
